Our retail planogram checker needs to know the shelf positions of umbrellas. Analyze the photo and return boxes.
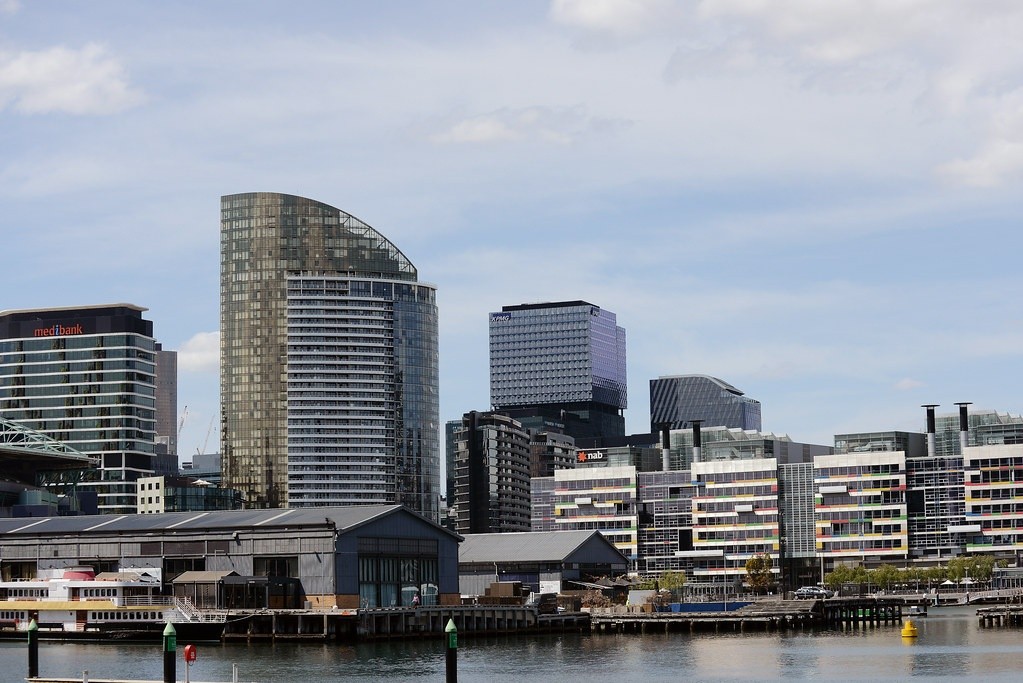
[960,578,973,588]
[940,580,955,588]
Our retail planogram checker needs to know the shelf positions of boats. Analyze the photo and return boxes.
[0,565,231,645]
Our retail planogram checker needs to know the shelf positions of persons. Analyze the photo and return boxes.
[412,593,419,609]
[923,592,926,601]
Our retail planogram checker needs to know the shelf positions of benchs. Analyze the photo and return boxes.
[37,622,64,631]
[0,622,17,632]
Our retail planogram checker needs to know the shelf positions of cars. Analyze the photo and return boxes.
[793,589,822,599]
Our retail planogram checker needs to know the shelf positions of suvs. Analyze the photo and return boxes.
[802,586,835,599]
